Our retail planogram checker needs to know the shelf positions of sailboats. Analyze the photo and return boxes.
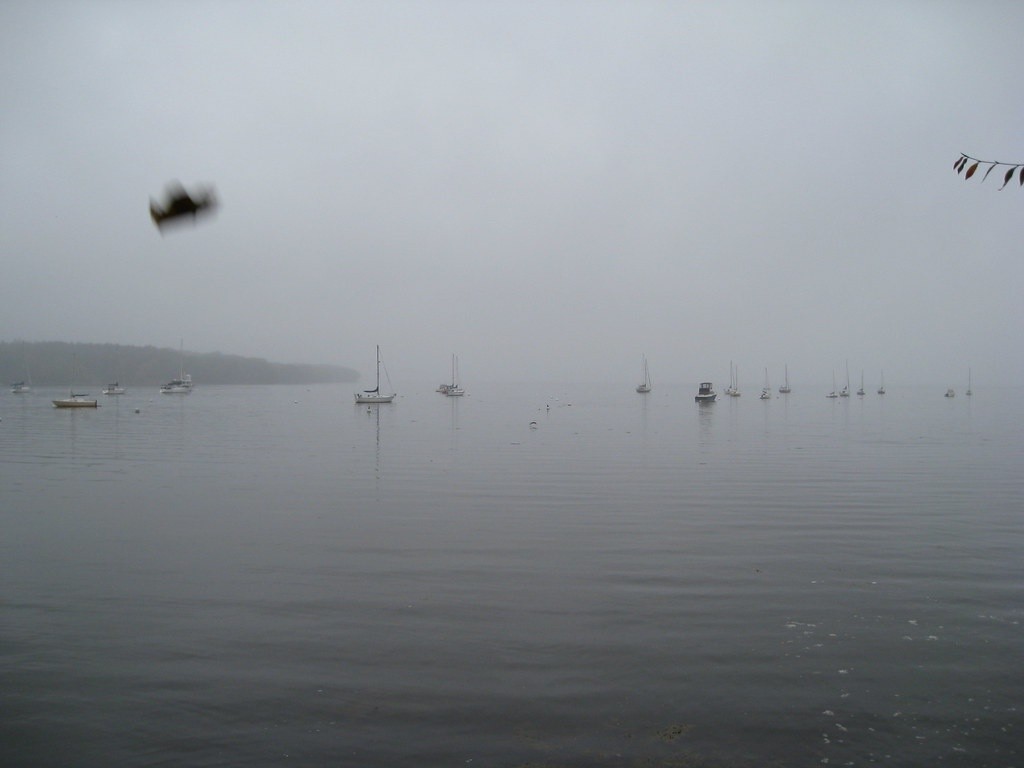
[855,368,867,396]
[876,369,887,394]
[636,356,652,393]
[725,360,741,397]
[840,362,851,397]
[436,354,467,396]
[759,367,772,400]
[353,344,398,403]
[777,362,793,394]
[825,368,838,399]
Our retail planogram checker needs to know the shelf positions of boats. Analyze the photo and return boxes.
[695,381,718,403]
[51,391,98,408]
[101,379,125,396]
[9,380,31,393]
[158,366,195,394]
